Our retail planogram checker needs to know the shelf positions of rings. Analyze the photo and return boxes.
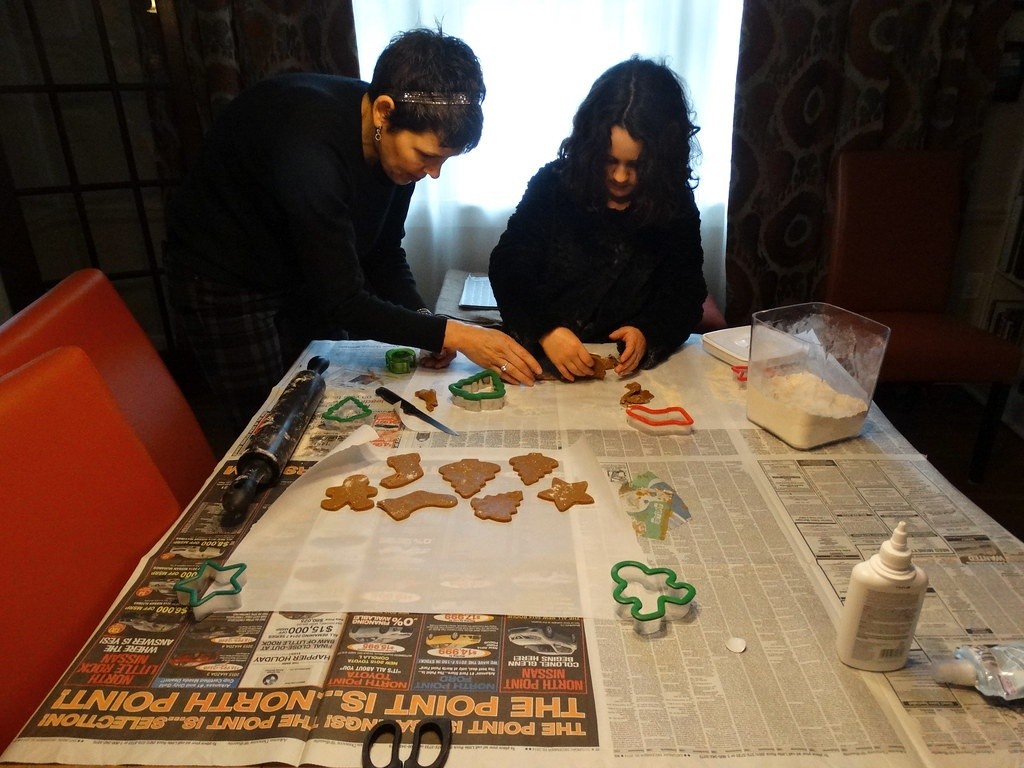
[500,363,508,372]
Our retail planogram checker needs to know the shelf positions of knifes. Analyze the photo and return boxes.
[375,387,460,438]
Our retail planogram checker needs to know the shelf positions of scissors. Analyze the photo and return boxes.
[361,717,453,768]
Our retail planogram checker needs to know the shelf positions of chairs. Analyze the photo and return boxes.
[1,267,211,755]
[829,148,1024,466]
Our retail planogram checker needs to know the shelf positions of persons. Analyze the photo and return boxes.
[488,58,707,384]
[162,28,543,461]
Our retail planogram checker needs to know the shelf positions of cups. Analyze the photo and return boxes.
[745,301,892,450]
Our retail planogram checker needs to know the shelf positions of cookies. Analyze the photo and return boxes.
[320,475,378,510]
[415,389,437,412]
[380,453,423,486]
[531,354,653,407]
[470,490,524,521]
[439,458,500,499]
[509,453,558,485]
[538,477,594,511]
[377,491,457,520]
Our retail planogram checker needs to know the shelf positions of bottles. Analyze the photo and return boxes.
[835,521,929,673]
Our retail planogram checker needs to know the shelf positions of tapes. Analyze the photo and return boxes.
[385,349,417,374]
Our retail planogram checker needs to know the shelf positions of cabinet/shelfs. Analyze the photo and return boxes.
[954,104,1024,437]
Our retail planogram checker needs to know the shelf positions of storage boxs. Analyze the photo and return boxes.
[748,303,893,451]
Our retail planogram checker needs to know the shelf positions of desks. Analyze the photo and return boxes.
[0,341,1024,768]
